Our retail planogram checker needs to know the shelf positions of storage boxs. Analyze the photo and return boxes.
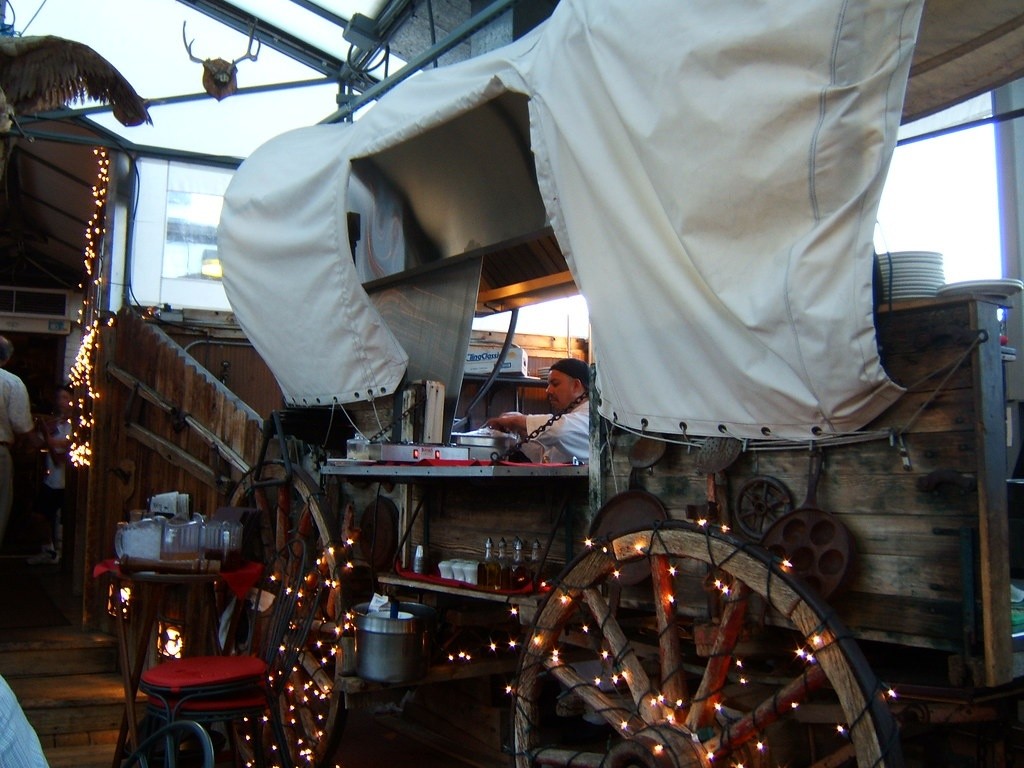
[465,347,527,376]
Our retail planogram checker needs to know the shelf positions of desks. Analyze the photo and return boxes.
[94,558,262,683]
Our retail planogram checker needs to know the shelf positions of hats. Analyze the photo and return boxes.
[549,358,589,389]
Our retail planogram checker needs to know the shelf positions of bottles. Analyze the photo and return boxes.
[413,545,424,574]
[346,433,369,460]
[478,536,547,593]
[437,557,479,586]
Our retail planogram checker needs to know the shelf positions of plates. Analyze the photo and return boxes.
[877,250,1023,304]
[329,459,376,467]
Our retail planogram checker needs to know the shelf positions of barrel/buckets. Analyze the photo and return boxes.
[351,600,436,683]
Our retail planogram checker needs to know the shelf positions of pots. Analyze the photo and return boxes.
[587,468,669,585]
[628,431,666,475]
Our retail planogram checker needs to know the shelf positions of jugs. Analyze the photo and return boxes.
[163,512,207,574]
[115,516,168,575]
[200,519,243,572]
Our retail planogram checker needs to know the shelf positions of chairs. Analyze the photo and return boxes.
[112,536,329,768]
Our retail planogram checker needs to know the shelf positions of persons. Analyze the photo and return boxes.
[482,358,592,465]
[1,337,68,570]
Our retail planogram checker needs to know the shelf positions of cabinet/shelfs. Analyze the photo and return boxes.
[315,463,591,608]
